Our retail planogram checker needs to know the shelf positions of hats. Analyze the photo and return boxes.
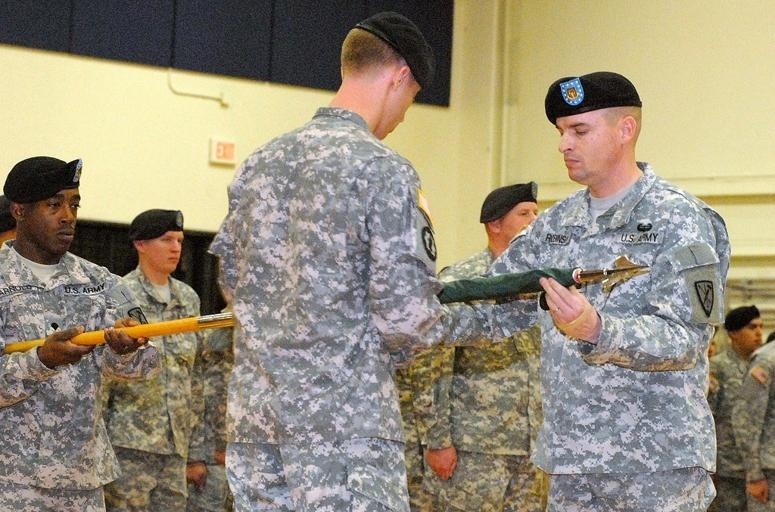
[354,11,437,90]
[480,181,538,223]
[545,72,642,125]
[129,209,183,240]
[725,306,760,331]
[3,156,83,203]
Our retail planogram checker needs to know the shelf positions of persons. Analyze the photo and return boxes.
[446,72,731,512]
[207,11,446,512]
